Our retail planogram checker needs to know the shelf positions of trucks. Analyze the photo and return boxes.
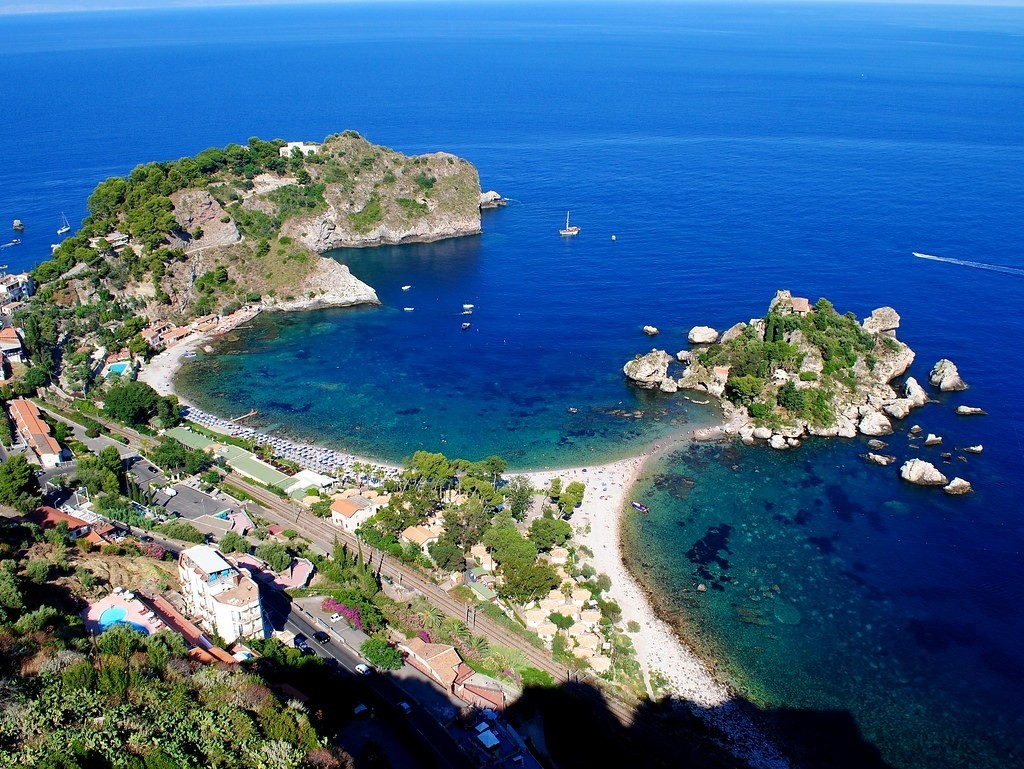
[163,487,177,497]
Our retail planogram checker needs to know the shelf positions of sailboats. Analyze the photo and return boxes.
[558,210,580,236]
[58,212,70,234]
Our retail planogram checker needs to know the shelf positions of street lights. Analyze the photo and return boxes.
[54,497,61,509]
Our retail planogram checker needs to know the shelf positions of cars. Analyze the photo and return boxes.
[313,630,331,644]
[295,639,307,650]
[148,466,158,474]
[330,613,344,622]
[141,535,154,542]
[131,456,144,462]
[303,646,318,657]
[397,700,414,716]
[355,664,371,677]
